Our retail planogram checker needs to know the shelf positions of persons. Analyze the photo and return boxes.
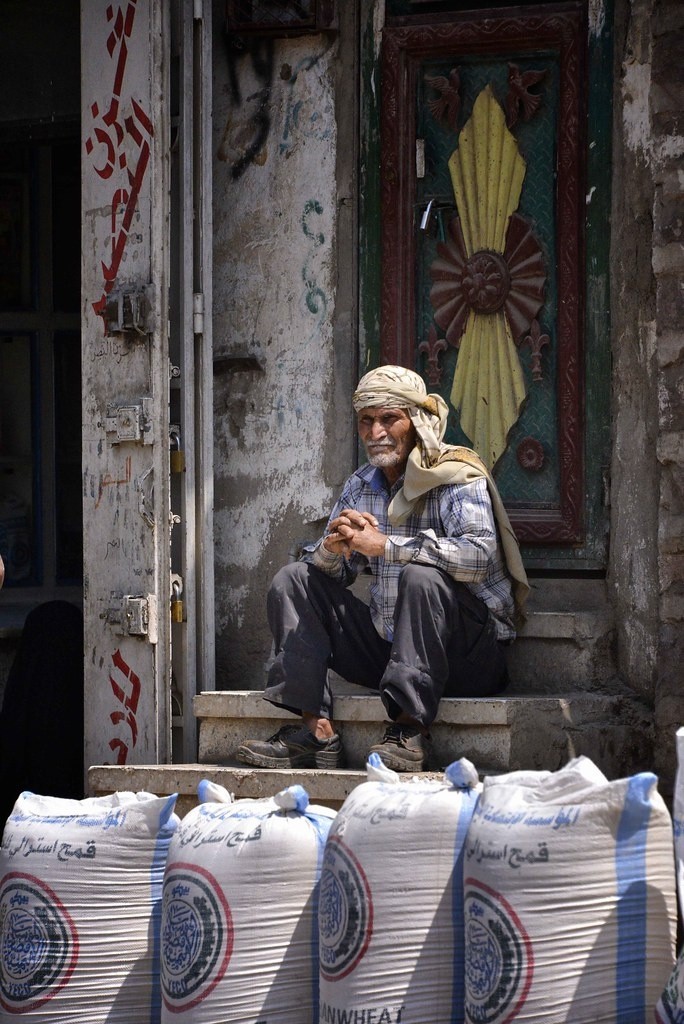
[236,365,521,770]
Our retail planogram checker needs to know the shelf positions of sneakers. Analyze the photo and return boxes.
[367,720,434,772]
[236,725,343,770]
